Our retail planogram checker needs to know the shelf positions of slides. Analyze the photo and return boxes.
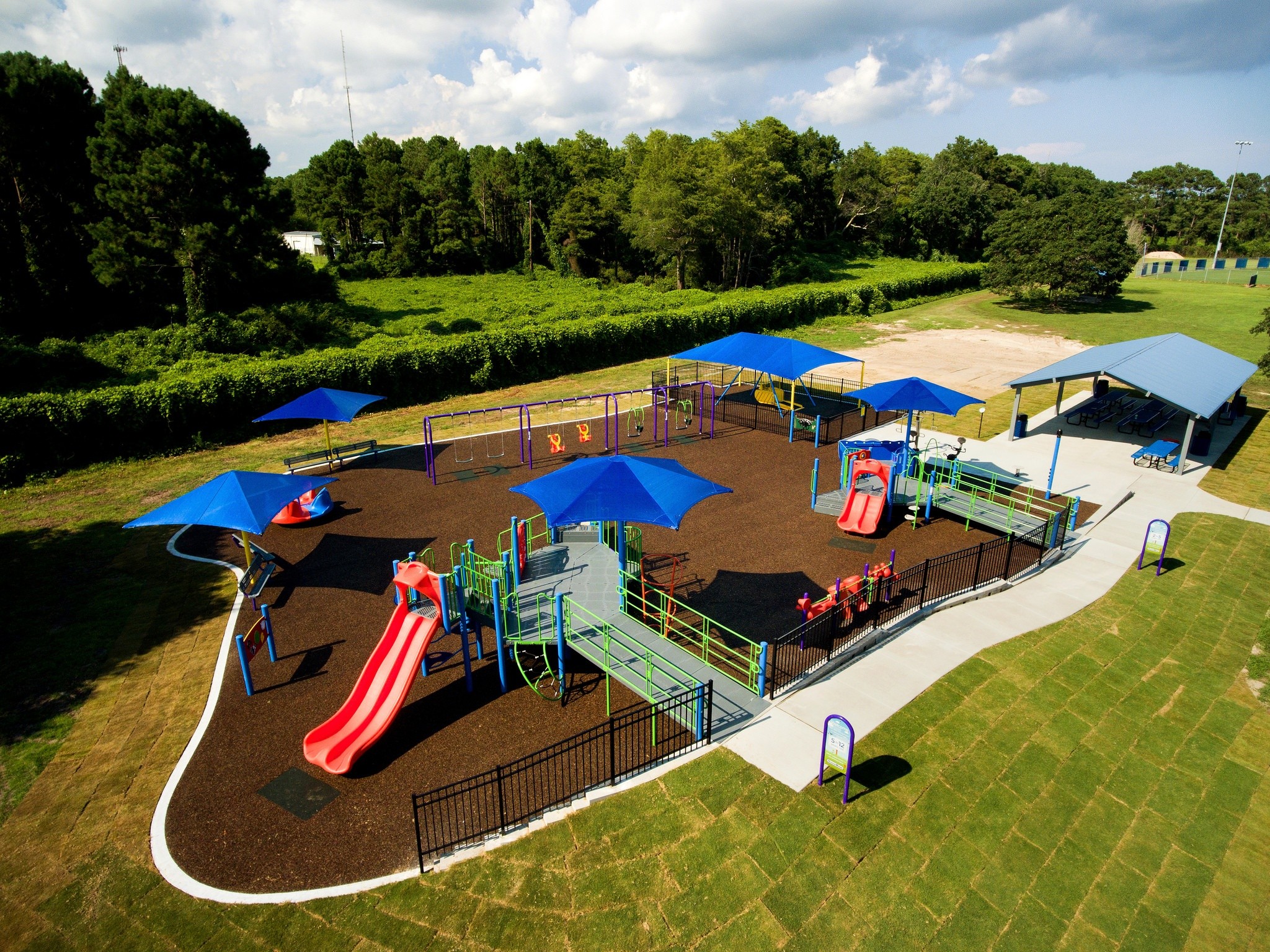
[837,459,890,535]
[302,561,443,776]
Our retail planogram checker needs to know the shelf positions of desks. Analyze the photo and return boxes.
[1144,439,1180,471]
[1141,400,1169,418]
[1128,409,1161,437]
[1101,390,1130,411]
[1077,401,1109,427]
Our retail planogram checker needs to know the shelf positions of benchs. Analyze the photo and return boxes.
[332,439,380,466]
[284,450,336,475]
[1131,446,1148,467]
[1064,396,1180,438]
[1167,453,1181,473]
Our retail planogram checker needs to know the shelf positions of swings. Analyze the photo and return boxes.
[626,391,645,438]
[674,385,693,430]
[545,402,566,454]
[575,398,592,443]
[483,409,505,458]
[451,414,474,463]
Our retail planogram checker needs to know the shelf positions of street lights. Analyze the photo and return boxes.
[1211,139,1254,269]
